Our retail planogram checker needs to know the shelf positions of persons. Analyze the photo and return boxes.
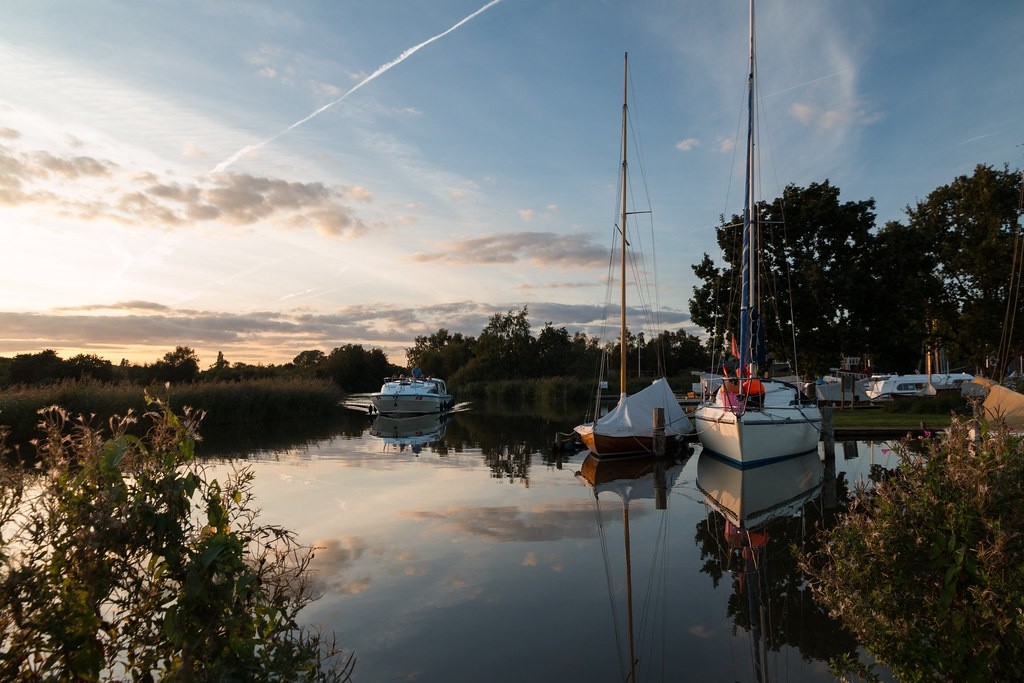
[392,363,431,382]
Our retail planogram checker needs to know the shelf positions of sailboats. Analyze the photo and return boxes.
[694,1,828,466]
[688,451,828,682]
[570,51,698,458]
[574,451,693,683]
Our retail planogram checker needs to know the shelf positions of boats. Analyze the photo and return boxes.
[369,413,448,445]
[370,366,454,414]
